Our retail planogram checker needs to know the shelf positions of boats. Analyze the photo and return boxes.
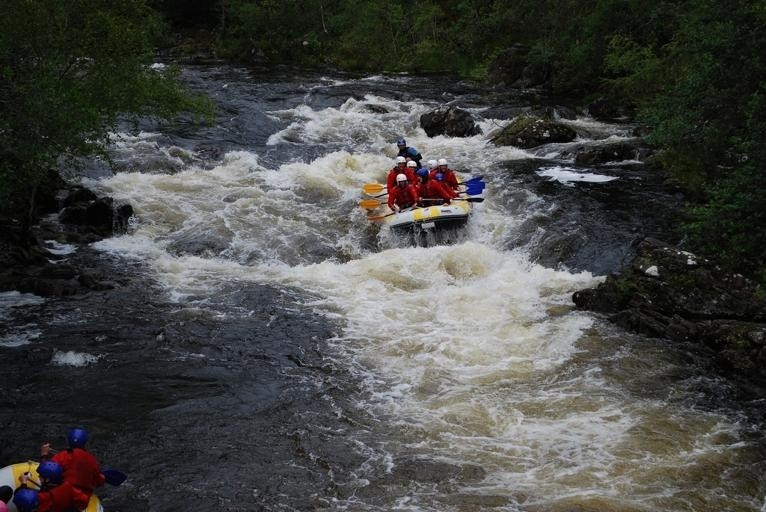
[1,458,104,512]
[375,174,471,230]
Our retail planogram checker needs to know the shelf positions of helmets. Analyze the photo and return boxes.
[38,462,63,481]
[398,139,406,146]
[13,488,39,511]
[396,156,428,186]
[68,428,89,448]
[428,159,448,181]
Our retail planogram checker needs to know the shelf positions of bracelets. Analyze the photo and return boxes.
[41,455,48,460]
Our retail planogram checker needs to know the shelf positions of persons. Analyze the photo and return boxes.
[15,460,88,510]
[39,425,106,493]
[13,487,50,511]
[387,138,461,214]
[0,485,12,511]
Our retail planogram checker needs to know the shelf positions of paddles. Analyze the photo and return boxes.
[422,198,484,202]
[50,448,127,486]
[457,176,485,195]
[359,184,395,220]
